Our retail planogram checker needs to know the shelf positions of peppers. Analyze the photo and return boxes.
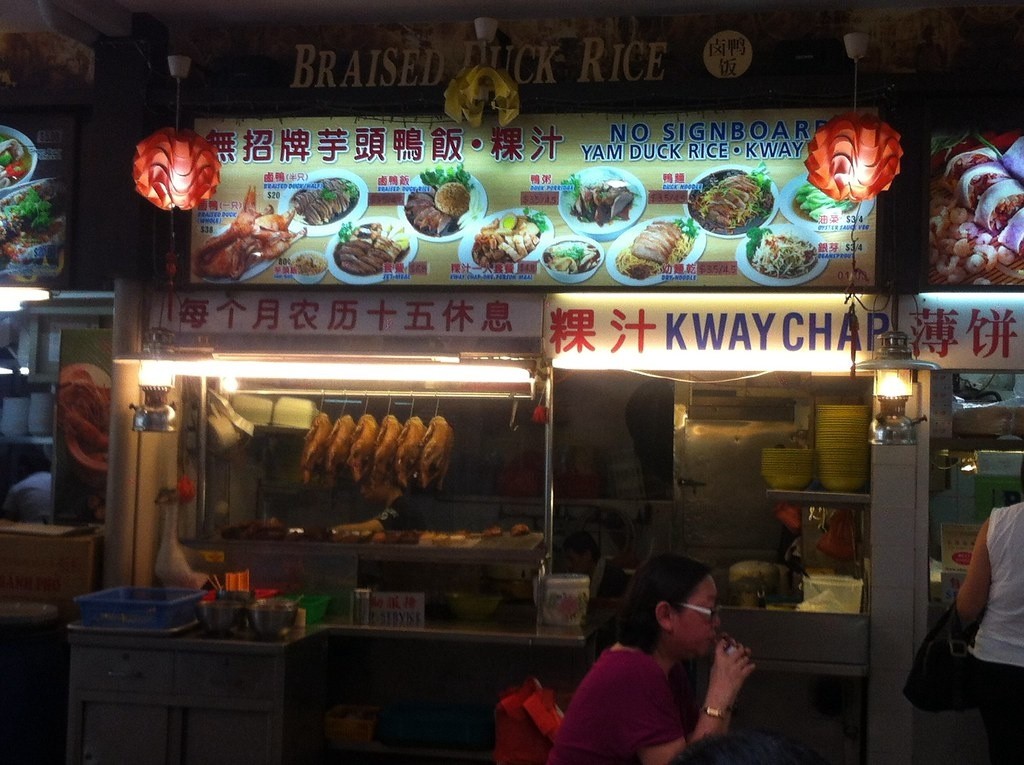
[0,134,27,178]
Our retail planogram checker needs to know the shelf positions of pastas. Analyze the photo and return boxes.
[616,234,690,280]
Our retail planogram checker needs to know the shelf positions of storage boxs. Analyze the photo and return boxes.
[73,584,208,629]
[976,449,1024,477]
[0,517,96,625]
[322,699,496,752]
[951,403,1024,437]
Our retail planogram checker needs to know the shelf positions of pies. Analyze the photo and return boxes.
[940,133,1023,257]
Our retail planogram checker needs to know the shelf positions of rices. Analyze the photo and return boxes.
[434,182,469,218]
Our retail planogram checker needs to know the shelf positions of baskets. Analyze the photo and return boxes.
[72,584,207,629]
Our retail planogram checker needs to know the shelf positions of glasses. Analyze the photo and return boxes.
[667,599,720,623]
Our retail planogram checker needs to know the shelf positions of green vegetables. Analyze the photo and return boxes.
[419,164,474,194]
[13,188,53,228]
[673,217,701,238]
[322,178,360,241]
[929,124,1005,161]
[701,171,856,259]
[521,174,622,262]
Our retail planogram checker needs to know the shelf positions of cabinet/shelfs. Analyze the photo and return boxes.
[322,595,625,765]
[64,632,291,765]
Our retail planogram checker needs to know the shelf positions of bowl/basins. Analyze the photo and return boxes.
[0,125,39,194]
[761,404,870,493]
[247,602,298,639]
[194,599,247,634]
[287,247,328,283]
[449,590,503,619]
[540,237,605,284]
[802,574,864,614]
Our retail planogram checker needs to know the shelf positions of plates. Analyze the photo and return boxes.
[195,166,874,285]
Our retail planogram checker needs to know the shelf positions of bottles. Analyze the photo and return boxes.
[543,573,589,627]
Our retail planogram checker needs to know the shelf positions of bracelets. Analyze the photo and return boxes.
[700,706,728,721]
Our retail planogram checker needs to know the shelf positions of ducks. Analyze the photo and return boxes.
[345,414,379,481]
[297,413,332,485]
[152,488,210,590]
[220,515,287,542]
[195,185,297,280]
[393,416,431,487]
[371,414,405,474]
[325,415,356,473]
[415,415,456,490]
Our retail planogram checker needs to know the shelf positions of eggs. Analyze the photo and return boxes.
[500,212,517,230]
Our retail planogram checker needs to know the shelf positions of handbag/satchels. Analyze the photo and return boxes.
[903,602,982,713]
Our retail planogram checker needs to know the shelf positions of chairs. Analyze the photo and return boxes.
[493,678,565,765]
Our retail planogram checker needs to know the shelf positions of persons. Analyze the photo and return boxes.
[4,456,52,524]
[955,457,1024,765]
[545,555,755,765]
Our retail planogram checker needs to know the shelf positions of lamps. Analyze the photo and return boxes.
[858,279,941,445]
[112,290,214,433]
